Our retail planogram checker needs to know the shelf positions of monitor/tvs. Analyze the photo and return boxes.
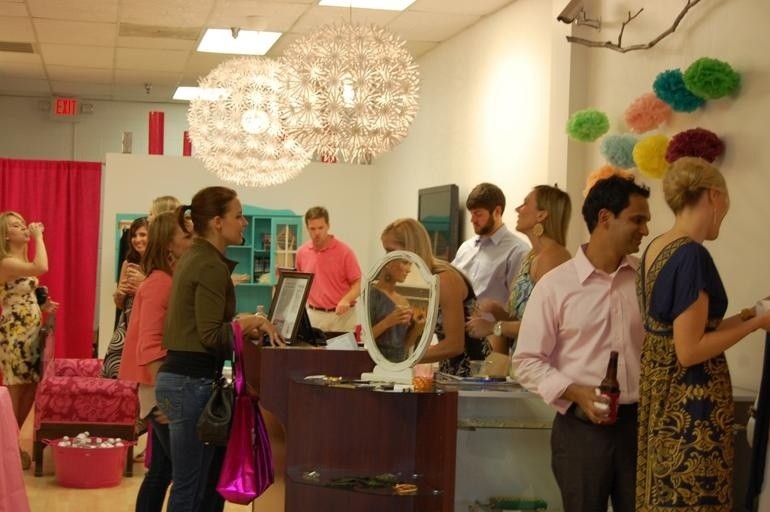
[418,184,459,262]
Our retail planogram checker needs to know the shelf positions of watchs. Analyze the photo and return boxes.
[493,320,504,337]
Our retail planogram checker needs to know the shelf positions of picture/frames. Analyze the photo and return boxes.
[266,272,314,345]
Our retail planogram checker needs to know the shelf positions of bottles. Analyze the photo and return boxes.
[25,221,45,234]
[599,351,621,426]
[254,255,269,280]
[58,431,125,449]
[253,304,270,319]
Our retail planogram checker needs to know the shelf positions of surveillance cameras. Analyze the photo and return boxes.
[557,0,585,24]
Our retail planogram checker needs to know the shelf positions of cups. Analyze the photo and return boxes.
[412,363,434,393]
[396,303,415,325]
[182,131,192,156]
[148,110,165,154]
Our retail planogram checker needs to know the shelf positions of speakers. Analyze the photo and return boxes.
[123,132,132,153]
[357,153,371,165]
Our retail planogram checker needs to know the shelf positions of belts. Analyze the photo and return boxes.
[309,304,356,312]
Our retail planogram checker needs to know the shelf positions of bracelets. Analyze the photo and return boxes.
[740,309,753,322]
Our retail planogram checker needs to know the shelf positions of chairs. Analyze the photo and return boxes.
[32,327,140,477]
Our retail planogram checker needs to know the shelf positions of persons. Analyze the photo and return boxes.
[510,168,651,511]
[295,206,362,332]
[368,257,426,364]
[634,154,770,512]
[448,182,532,322]
[98,188,289,512]
[0,211,60,470]
[464,184,573,384]
[381,218,492,377]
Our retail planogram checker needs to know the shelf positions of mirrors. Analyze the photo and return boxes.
[360,250,441,385]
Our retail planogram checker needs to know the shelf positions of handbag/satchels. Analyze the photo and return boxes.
[215,395,275,506]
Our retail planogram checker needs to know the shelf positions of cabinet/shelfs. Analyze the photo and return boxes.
[241,336,373,512]
[116,206,301,313]
[434,372,759,512]
[286,379,459,512]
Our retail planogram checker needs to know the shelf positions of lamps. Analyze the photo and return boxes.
[268,5,420,165]
[187,55,311,187]
